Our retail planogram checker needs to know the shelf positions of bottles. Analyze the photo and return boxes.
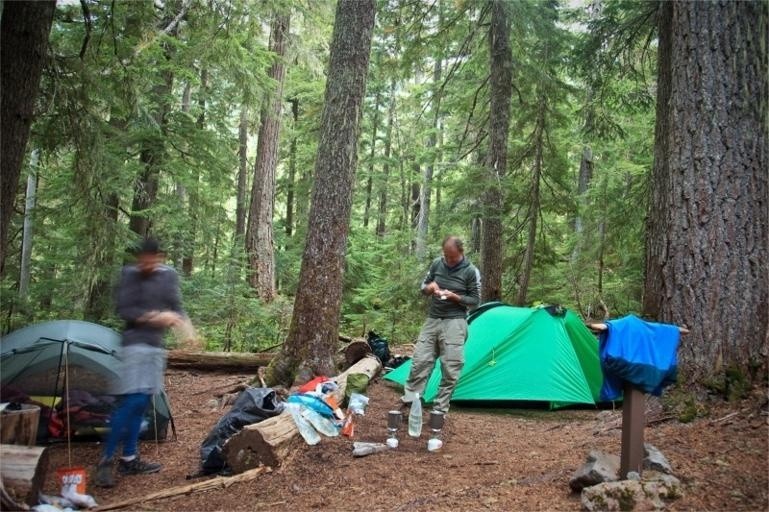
[386,411,402,447]
[408,393,422,437]
[428,408,444,452]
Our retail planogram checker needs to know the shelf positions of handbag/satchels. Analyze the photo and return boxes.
[367,330,390,360]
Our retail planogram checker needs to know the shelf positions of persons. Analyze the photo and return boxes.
[91,236,186,487]
[396,233,484,420]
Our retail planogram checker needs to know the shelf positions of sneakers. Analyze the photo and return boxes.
[118,456,160,476]
[91,461,119,488]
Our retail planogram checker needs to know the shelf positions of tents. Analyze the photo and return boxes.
[377,296,626,413]
[0,318,173,443]
